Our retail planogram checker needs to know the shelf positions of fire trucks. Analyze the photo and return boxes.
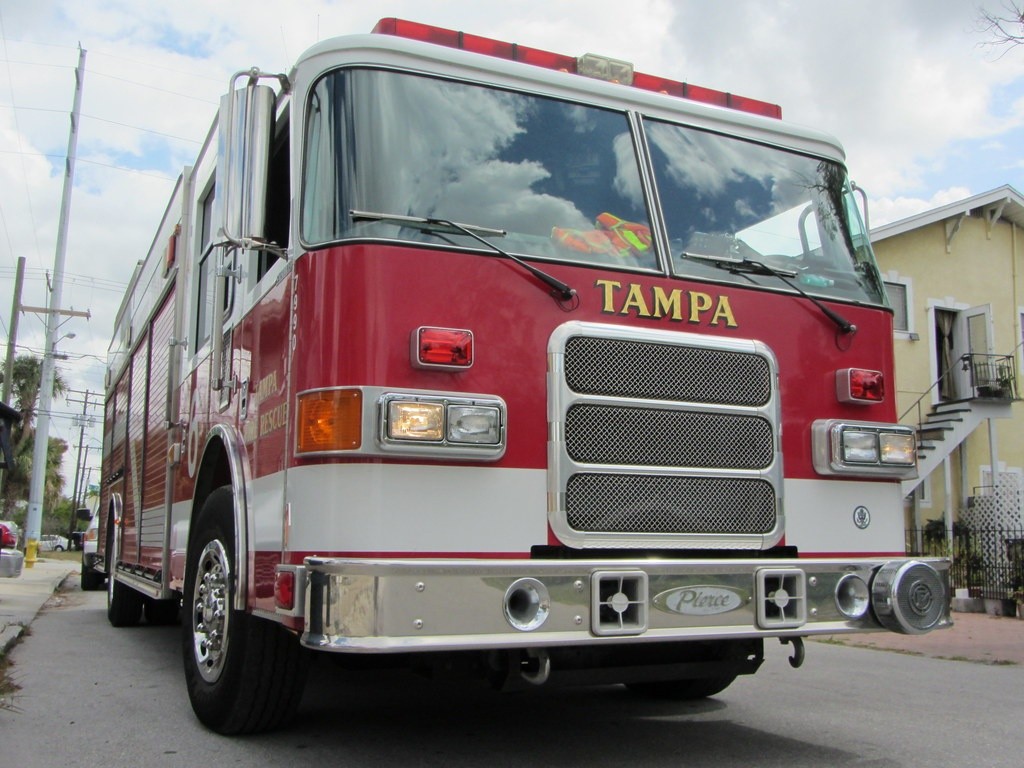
[92,17,955,737]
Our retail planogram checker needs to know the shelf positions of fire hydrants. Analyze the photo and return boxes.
[24,537,42,567]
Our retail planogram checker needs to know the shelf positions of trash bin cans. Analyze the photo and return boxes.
[0,400,24,501]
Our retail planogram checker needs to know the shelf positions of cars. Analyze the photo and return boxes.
[36,534,76,553]
[75,502,105,590]
[62,532,86,548]
